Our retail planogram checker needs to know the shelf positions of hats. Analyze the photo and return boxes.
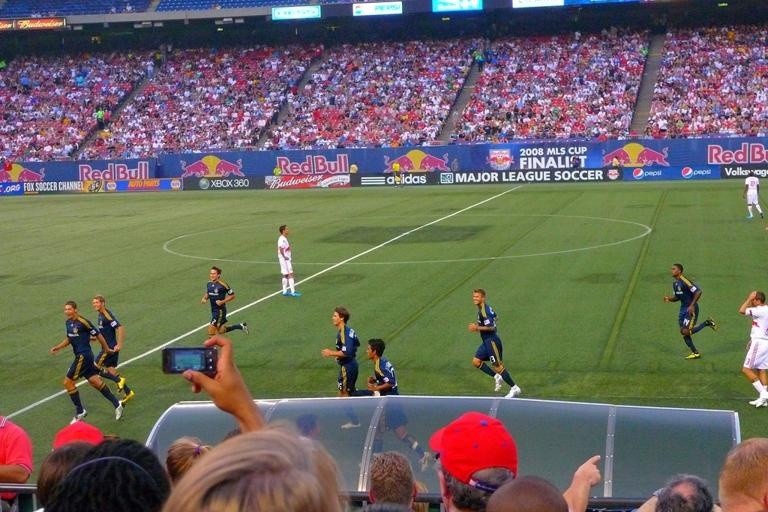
[429,411,518,493]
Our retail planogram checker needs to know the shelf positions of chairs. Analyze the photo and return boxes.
[1,0,379,17]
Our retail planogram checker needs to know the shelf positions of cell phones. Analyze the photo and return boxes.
[162,345,217,374]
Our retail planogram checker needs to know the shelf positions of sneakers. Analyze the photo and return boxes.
[495,375,503,391]
[282,291,302,296]
[684,353,700,359]
[421,452,429,471]
[71,409,86,424]
[240,321,248,334]
[505,385,520,398]
[749,391,768,407]
[706,317,717,332]
[114,377,134,420]
[341,423,360,429]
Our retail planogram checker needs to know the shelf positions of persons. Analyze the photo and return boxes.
[319,308,383,429]
[467,288,522,400]
[48,301,127,429]
[89,295,137,404]
[209,2,222,10]
[506,24,653,142]
[122,1,135,12]
[30,9,57,17]
[109,6,120,14]
[663,263,720,361]
[1,333,768,511]
[649,14,674,35]
[742,171,765,221]
[0,51,162,164]
[651,10,768,139]
[156,40,325,154]
[200,267,251,339]
[738,290,768,409]
[320,22,511,148]
[277,223,302,297]
